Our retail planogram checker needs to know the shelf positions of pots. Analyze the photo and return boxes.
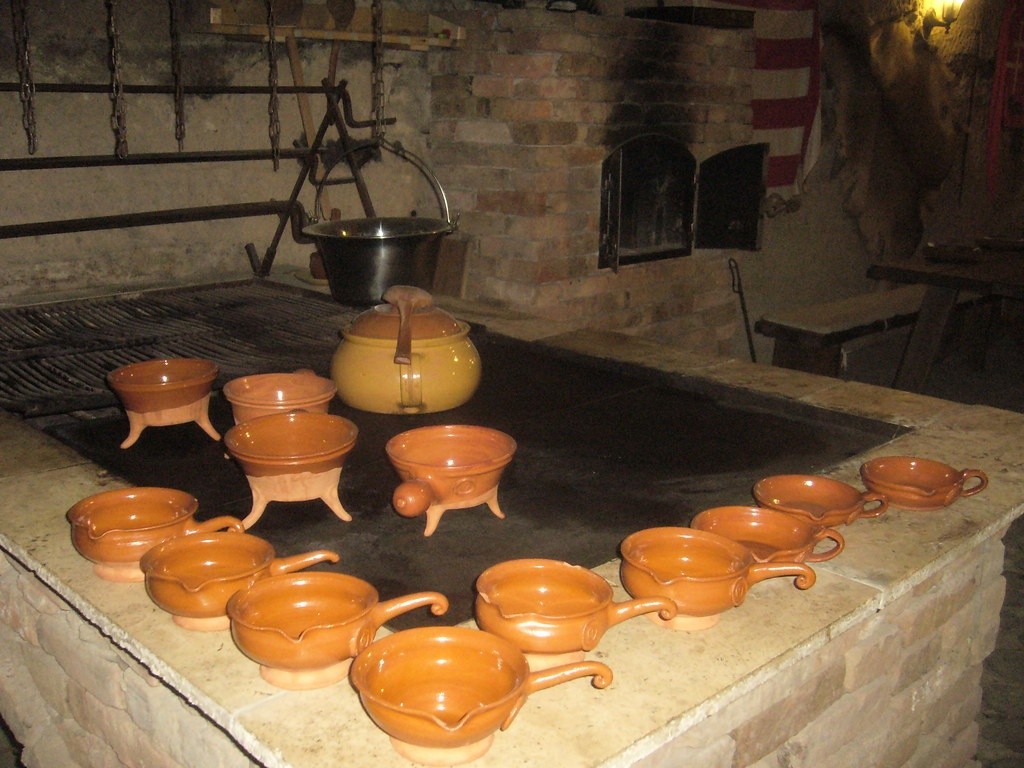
[330,284,482,417]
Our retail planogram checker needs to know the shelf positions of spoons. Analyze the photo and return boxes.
[383,285,434,364]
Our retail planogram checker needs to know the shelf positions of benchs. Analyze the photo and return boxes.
[755,287,992,378]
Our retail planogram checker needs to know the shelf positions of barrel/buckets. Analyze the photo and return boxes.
[302,132,461,309]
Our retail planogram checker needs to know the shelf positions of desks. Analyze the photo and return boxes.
[868,251,1024,393]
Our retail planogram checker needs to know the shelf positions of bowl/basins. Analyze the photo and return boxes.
[226,573,448,690]
[619,527,817,630]
[224,410,359,530]
[475,558,678,670]
[385,425,517,537]
[860,456,988,510]
[350,626,613,768]
[107,359,222,448]
[224,369,337,425]
[139,532,340,632]
[66,487,245,583]
[752,475,888,528]
[691,506,845,565]
